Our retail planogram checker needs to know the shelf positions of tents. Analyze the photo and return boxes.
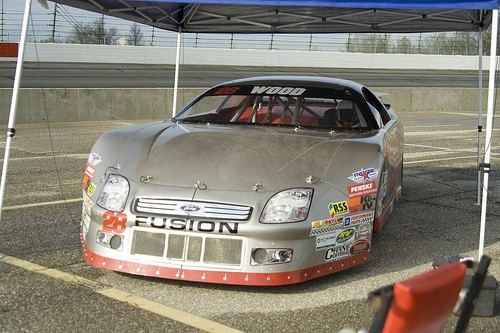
[1,0,500,268]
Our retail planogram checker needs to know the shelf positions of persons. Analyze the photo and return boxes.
[331,98,362,127]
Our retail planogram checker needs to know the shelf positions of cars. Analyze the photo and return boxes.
[78,77,406,286]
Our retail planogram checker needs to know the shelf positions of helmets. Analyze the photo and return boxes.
[335,100,359,128]
